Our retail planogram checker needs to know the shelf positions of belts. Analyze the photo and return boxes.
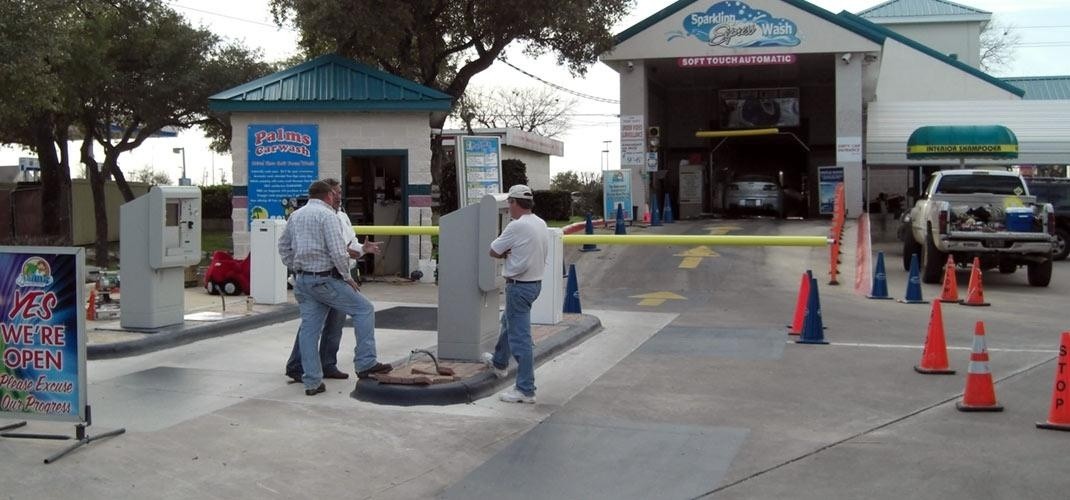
[506,279,541,284]
[297,269,330,276]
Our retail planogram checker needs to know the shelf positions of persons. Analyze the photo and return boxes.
[277,181,392,396]
[481,185,550,405]
[282,176,387,384]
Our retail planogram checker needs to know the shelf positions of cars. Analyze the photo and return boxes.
[724,172,788,221]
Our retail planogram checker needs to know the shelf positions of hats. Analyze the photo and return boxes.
[509,184,533,200]
[309,181,336,195]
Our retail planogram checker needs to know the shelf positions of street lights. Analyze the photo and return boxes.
[173,147,186,179]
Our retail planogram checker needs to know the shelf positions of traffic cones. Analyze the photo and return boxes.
[661,192,674,223]
[784,268,831,348]
[86,288,97,321]
[562,264,583,315]
[648,192,664,227]
[578,211,602,253]
[865,250,894,300]
[1035,331,1069,432]
[614,202,627,235]
[899,253,930,306]
[643,202,651,224]
[959,257,992,307]
[912,296,957,376]
[939,254,965,304]
[956,320,1007,413]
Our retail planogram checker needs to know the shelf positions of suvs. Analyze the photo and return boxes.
[896,175,1069,260]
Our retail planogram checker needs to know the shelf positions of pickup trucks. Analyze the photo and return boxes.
[895,169,1061,288]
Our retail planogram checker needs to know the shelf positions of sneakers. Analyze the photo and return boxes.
[482,352,508,380]
[501,387,536,404]
[356,364,392,380]
[287,371,304,382]
[325,369,348,379]
[306,384,325,395]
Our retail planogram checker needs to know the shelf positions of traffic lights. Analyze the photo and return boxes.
[648,126,660,152]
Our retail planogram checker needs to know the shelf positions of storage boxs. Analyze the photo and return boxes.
[1004,207,1033,232]
